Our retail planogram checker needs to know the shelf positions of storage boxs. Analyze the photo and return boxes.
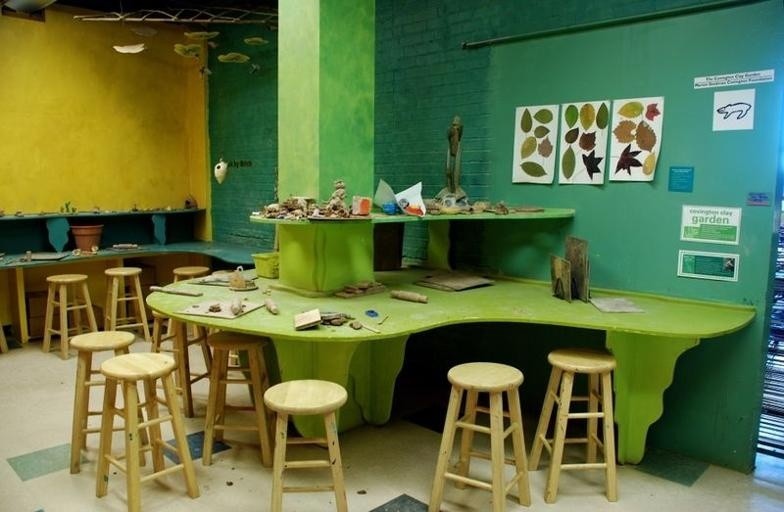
[26,289,60,317]
[28,317,60,337]
[68,304,103,331]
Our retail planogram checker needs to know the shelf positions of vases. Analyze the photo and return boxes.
[70,224,104,252]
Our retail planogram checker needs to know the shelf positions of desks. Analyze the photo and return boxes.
[0,242,273,343]
[145,262,759,466]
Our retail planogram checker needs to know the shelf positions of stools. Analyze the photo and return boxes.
[263,378,349,512]
[102,267,151,346]
[69,330,149,475]
[212,269,241,368]
[95,352,200,512]
[167,266,211,348]
[149,308,215,418]
[202,330,277,468]
[529,346,619,502]
[41,273,98,361]
[424,361,531,511]
